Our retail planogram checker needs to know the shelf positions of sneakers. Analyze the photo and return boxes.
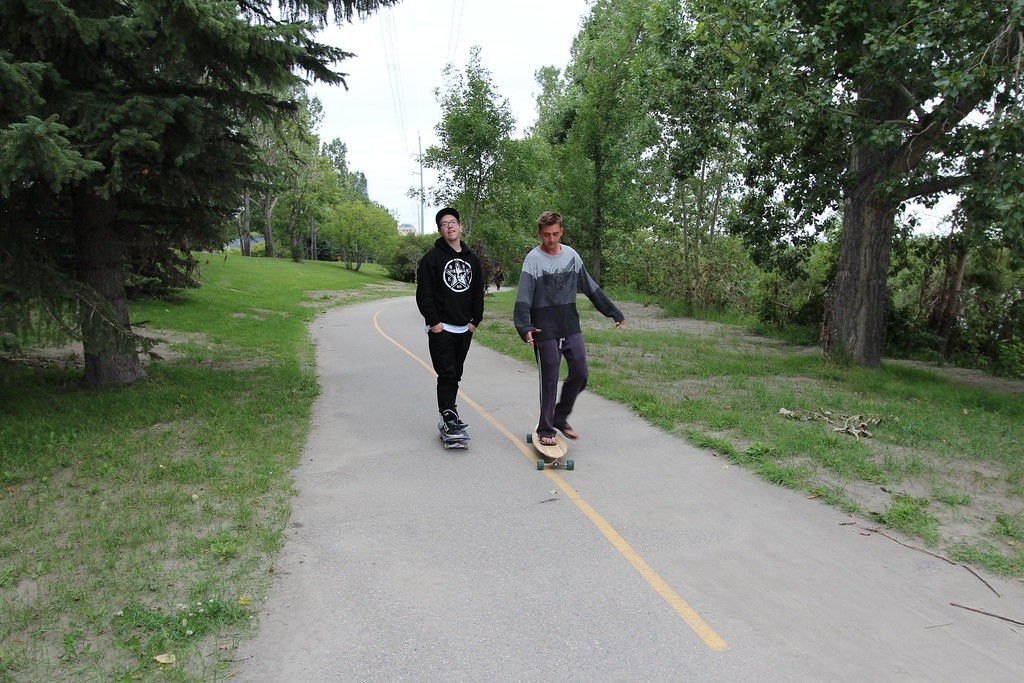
[438,405,469,437]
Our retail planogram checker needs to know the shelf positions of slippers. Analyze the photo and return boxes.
[553,421,578,439]
[538,433,557,446]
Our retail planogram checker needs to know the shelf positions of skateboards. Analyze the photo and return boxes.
[526,429,575,471]
[439,422,470,452]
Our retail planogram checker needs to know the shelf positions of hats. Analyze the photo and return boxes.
[435,207,459,223]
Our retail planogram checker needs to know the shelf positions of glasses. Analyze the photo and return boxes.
[440,220,458,227]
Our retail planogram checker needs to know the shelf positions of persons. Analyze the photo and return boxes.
[494,269,504,291]
[513,212,624,444]
[416,209,483,437]
